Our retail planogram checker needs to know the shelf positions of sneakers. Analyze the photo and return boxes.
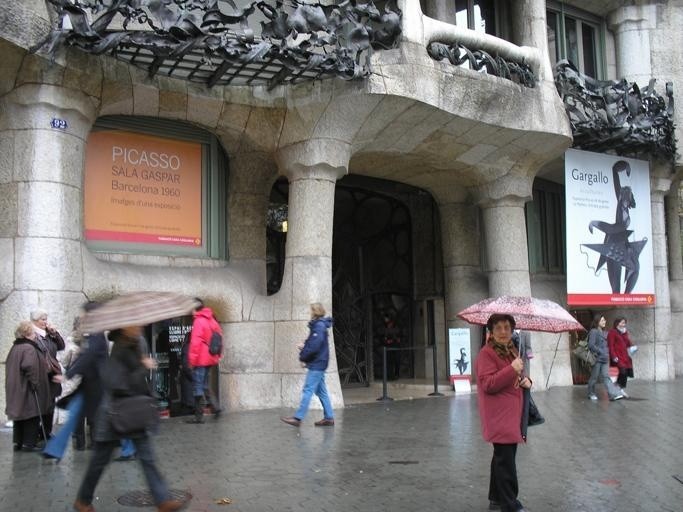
[620,389,629,399]
[39,433,52,440]
[21,443,42,452]
[155,495,188,512]
[113,454,137,462]
[314,415,334,427]
[278,414,302,428]
[488,498,502,509]
[74,445,85,451]
[72,496,97,512]
[212,410,223,420]
[37,451,61,464]
[500,505,531,512]
[87,442,94,450]
[11,443,20,451]
[183,417,205,424]
[586,393,597,400]
[608,395,624,401]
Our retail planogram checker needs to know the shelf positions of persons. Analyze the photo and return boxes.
[280,302,334,426]
[5,312,150,465]
[607,316,638,398]
[573,314,624,401]
[384,313,402,379]
[186,297,224,423]
[75,326,186,512]
[475,314,543,512]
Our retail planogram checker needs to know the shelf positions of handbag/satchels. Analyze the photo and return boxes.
[44,352,62,375]
[572,340,597,366]
[108,395,157,434]
[207,331,222,355]
[527,390,545,427]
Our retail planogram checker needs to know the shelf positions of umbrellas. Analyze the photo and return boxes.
[457,295,588,374]
[75,291,204,380]
